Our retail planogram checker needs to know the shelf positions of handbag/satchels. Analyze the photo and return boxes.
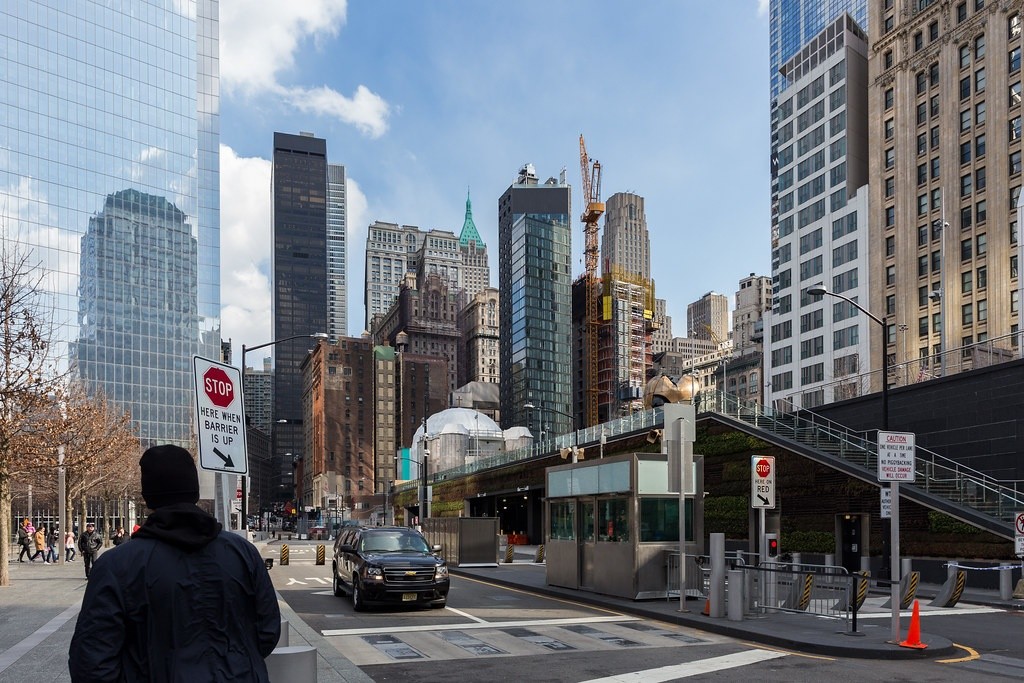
[17,537,25,545]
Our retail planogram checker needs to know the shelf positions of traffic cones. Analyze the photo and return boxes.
[700,593,710,616]
[900,599,929,649]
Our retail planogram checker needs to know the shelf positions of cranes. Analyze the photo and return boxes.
[579,133,606,428]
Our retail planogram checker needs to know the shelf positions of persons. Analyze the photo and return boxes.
[65,532,77,561]
[113,527,131,546]
[68,444,282,683]
[30,526,51,565]
[17,521,35,562]
[402,537,410,546]
[78,523,103,580]
[46,527,58,563]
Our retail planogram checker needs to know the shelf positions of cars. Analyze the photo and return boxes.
[54,525,79,540]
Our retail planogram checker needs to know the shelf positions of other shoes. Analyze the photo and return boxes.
[66,560,71,562]
[28,560,32,564]
[44,561,49,564]
[17,558,24,562]
[71,558,75,561]
[52,561,58,563]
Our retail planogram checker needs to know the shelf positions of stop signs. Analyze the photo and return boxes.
[203,366,235,409]
[756,459,771,477]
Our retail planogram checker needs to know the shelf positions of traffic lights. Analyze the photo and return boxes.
[768,538,777,557]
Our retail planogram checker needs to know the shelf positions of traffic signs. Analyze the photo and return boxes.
[191,353,249,477]
[751,477,776,510]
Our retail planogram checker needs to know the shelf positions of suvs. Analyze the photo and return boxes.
[331,524,450,612]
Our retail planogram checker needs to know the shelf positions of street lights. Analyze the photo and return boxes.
[361,478,386,525]
[242,333,328,393]
[392,456,424,485]
[259,452,294,531]
[522,402,579,447]
[807,283,896,587]
